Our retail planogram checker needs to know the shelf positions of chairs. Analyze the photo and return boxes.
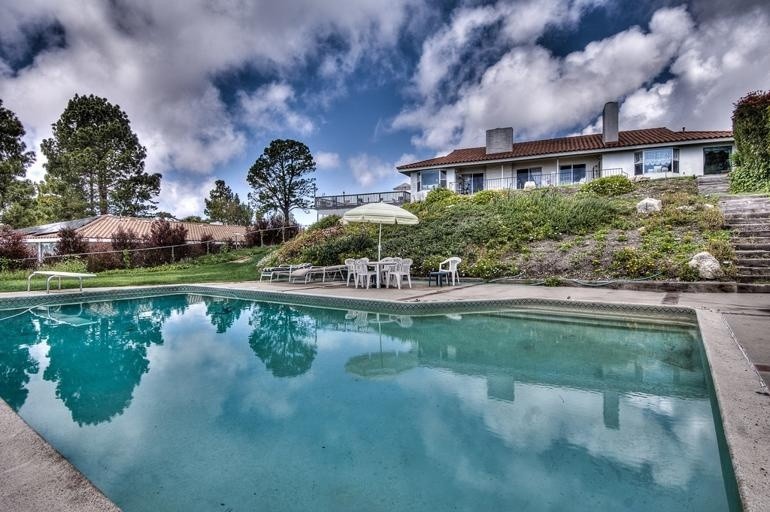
[346,310,461,333]
[260,256,462,290]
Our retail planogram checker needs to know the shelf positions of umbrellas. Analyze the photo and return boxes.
[343,199,420,261]
[342,321,420,383]
